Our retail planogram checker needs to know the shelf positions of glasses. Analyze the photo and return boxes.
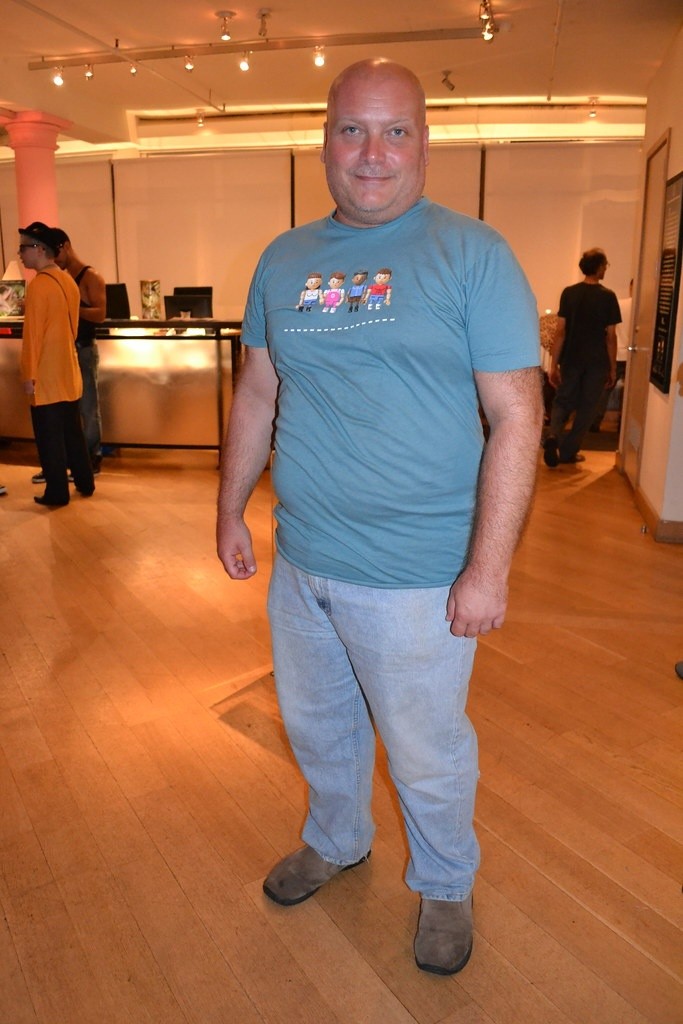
[19,243,39,252]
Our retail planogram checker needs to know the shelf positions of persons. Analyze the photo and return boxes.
[31,227,106,484]
[589,278,635,434]
[17,221,96,506]
[543,247,623,468]
[211,55,544,973]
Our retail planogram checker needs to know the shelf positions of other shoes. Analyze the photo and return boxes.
[542,437,584,467]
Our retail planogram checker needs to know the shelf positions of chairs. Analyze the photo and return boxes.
[163,295,213,320]
[105,282,131,319]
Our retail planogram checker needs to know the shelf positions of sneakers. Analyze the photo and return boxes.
[413,893,475,974]
[31,471,46,483]
[262,841,370,905]
[68,471,98,482]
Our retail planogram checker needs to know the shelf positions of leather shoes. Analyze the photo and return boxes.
[34,494,69,507]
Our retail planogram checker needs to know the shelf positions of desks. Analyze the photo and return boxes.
[0,319,244,472]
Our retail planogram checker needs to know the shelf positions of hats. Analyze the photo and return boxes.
[18,221,61,255]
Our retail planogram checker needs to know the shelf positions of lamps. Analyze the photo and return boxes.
[588,97,600,118]
[84,64,93,81]
[240,50,253,72]
[54,66,64,86]
[130,64,136,74]
[183,55,194,70]
[479,0,496,41]
[196,109,207,128]
[214,10,237,40]
[254,8,271,37]
[313,44,326,67]
